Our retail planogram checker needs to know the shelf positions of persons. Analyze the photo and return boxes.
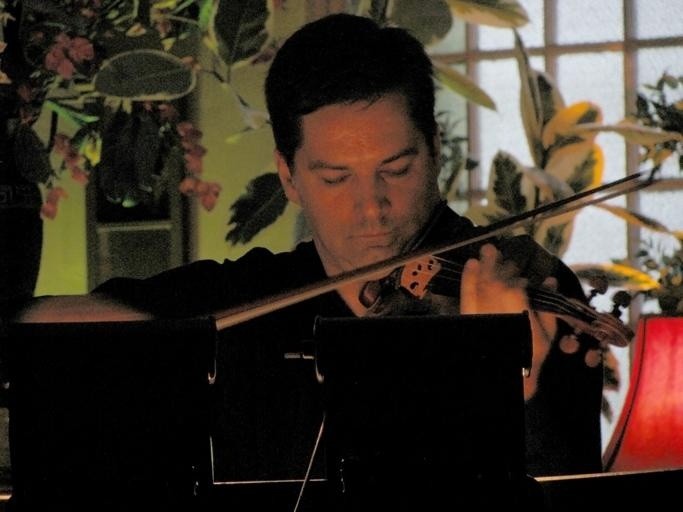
[7,11,606,478]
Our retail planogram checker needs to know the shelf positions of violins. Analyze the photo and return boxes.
[360,200,634,369]
